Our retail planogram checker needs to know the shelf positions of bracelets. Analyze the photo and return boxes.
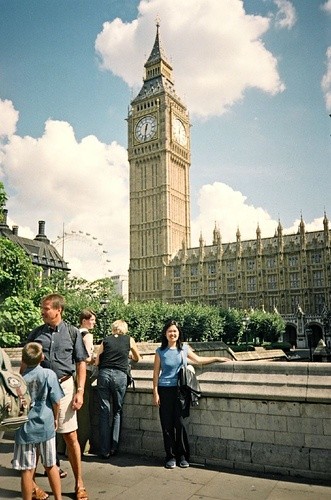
[76,387,85,394]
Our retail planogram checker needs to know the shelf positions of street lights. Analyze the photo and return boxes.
[100,289,111,338]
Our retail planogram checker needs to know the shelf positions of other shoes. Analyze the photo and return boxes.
[165,456,177,469]
[98,447,118,458]
[176,455,189,467]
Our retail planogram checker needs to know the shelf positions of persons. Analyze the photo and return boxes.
[94,319,140,460]
[153,320,233,467]
[0,294,96,500]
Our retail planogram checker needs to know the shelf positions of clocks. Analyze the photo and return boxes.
[134,115,158,143]
[173,119,187,145]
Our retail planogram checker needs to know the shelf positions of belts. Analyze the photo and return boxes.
[59,374,72,383]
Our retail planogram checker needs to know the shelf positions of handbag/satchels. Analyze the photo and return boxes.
[127,373,136,391]
[84,364,99,387]
[176,368,188,392]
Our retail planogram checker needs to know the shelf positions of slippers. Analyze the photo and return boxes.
[45,468,66,478]
[75,486,89,500]
[21,486,49,500]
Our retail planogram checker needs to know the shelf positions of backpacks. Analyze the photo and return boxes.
[0,349,32,433]
